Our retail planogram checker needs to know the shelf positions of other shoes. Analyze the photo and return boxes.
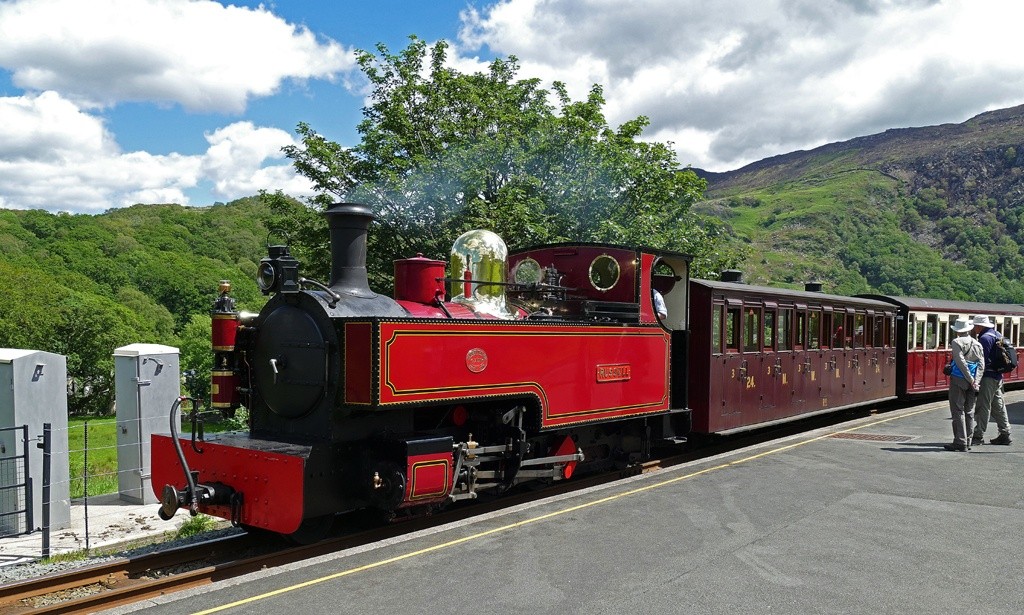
[944,443,968,452]
[990,434,1012,445]
[972,437,984,445]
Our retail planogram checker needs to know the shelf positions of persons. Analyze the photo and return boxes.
[969,315,1013,445]
[833,326,845,348]
[944,315,985,452]
[653,289,667,320]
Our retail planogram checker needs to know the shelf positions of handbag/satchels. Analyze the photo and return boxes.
[943,364,952,375]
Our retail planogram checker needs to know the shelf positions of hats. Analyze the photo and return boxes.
[973,315,994,328]
[950,319,974,333]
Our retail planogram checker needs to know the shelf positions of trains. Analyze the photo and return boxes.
[151,203,1024,539]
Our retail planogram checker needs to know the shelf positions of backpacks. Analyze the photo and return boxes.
[984,332,1017,373]
